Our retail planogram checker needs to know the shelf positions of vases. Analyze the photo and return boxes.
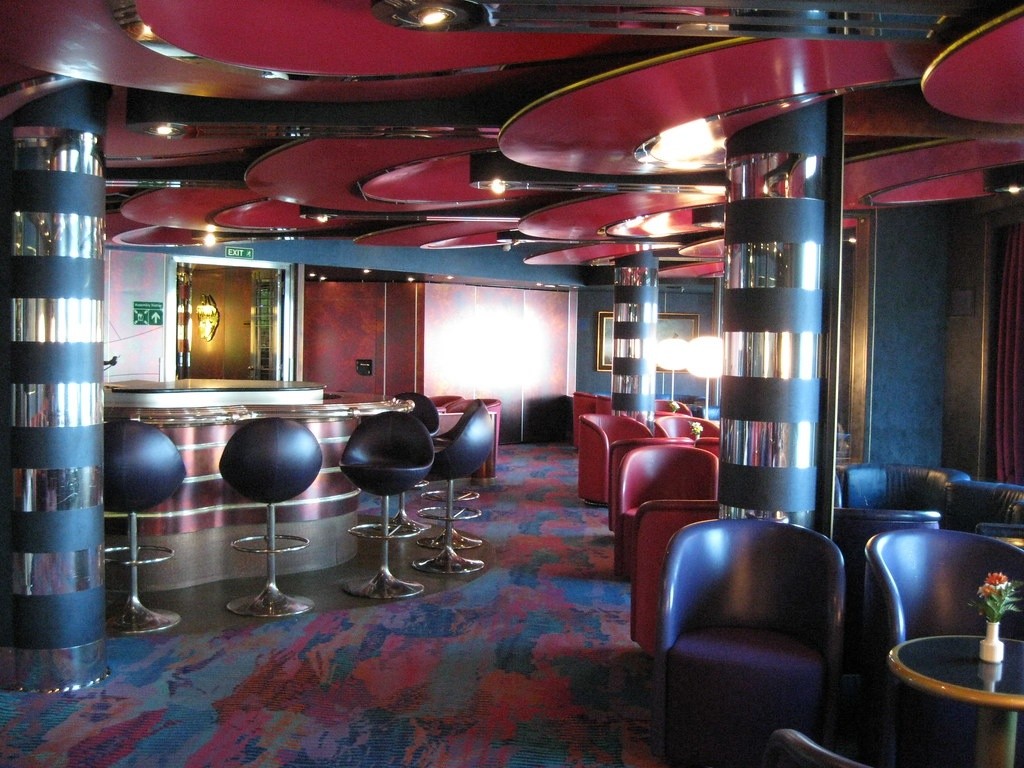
[978,663,1002,692]
[979,620,1005,664]
[696,434,700,440]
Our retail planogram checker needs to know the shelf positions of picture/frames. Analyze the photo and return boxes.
[596,310,700,373]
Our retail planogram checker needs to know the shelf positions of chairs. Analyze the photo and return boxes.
[696,437,720,458]
[860,528,1024,768]
[651,519,847,768]
[573,391,596,448]
[655,399,692,417]
[834,509,941,674]
[446,399,502,479]
[690,407,703,417]
[609,437,694,532]
[654,416,720,440]
[614,444,719,577]
[578,413,653,506]
[701,405,720,420]
[655,394,699,404]
[761,729,873,768]
[596,395,611,413]
[944,479,1024,533]
[695,398,711,405]
[843,463,971,513]
[428,395,464,410]
[630,499,719,657]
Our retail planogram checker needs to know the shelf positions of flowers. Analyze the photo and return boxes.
[668,401,680,412]
[967,571,1024,623]
[689,421,703,435]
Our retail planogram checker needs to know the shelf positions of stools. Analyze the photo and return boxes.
[104,419,186,635]
[219,417,323,618]
[412,399,494,574]
[416,489,483,550]
[339,410,435,600]
[377,392,440,530]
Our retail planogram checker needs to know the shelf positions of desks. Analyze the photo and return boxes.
[887,636,1024,768]
